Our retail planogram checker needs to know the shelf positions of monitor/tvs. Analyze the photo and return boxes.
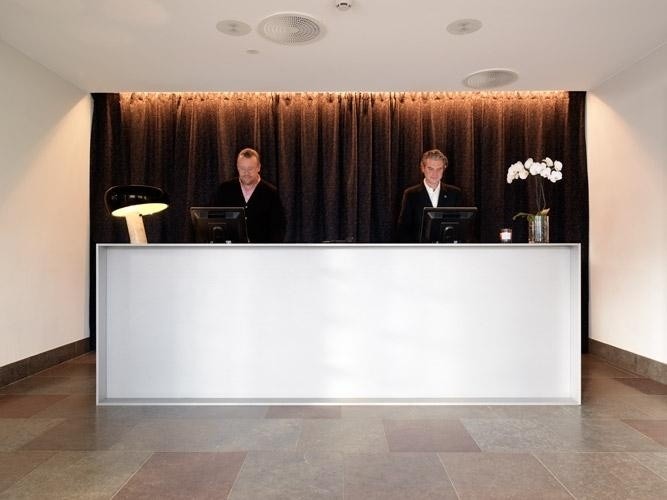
[190,207,249,243]
[421,207,477,244]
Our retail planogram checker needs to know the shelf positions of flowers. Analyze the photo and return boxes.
[507,157,563,226]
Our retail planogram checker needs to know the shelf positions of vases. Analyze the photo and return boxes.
[528,215,550,243]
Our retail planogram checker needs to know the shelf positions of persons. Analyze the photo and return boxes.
[395,148,468,243]
[210,147,287,243]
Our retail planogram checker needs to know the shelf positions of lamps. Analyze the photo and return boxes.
[104,184,169,244]
